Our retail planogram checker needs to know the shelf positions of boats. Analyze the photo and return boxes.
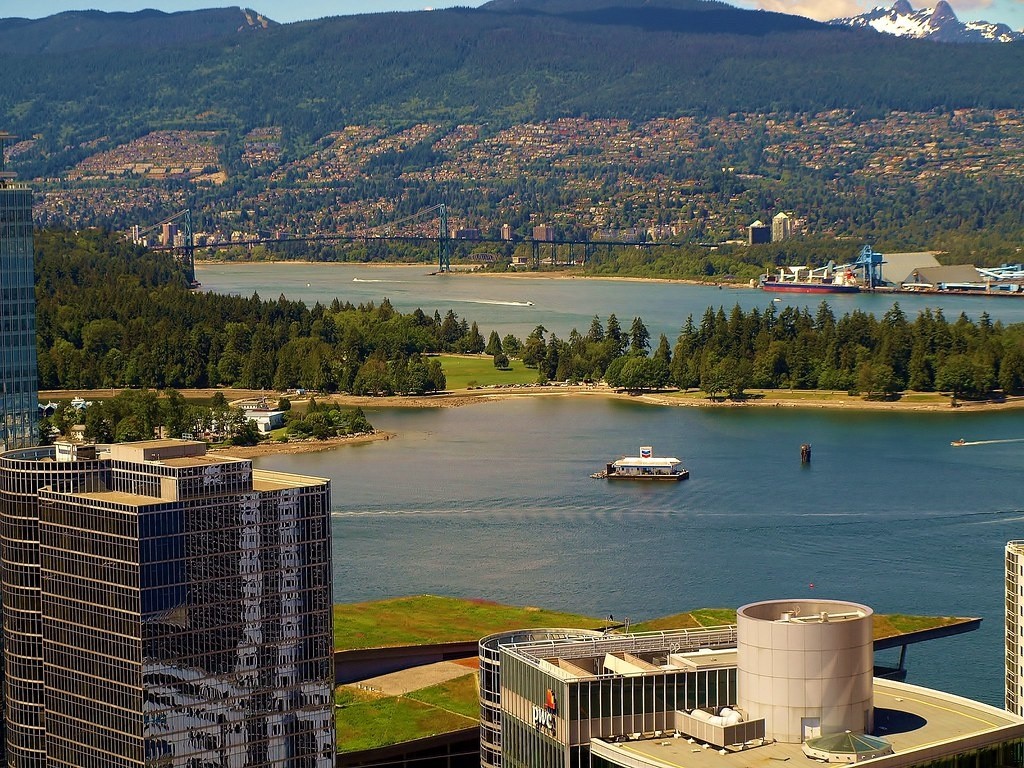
[763,269,861,294]
[950,439,966,446]
[589,447,690,481]
